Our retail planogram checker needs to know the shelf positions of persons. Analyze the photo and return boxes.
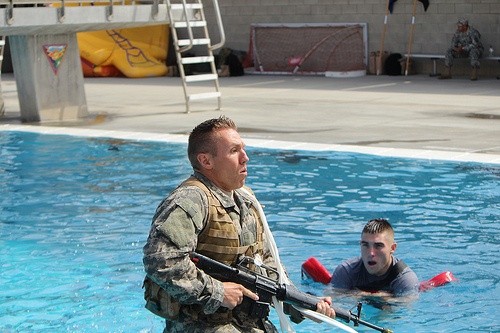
[438,17,485,80]
[329,219,419,309]
[142,116,336,333]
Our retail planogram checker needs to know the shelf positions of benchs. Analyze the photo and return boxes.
[404,54,447,78]
[452,57,500,80]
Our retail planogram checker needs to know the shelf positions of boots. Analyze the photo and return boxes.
[471,68,478,80]
[439,66,450,79]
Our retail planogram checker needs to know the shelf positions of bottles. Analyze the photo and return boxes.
[489,46,494,56]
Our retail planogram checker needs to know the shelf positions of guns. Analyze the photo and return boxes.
[189,252,394,332]
